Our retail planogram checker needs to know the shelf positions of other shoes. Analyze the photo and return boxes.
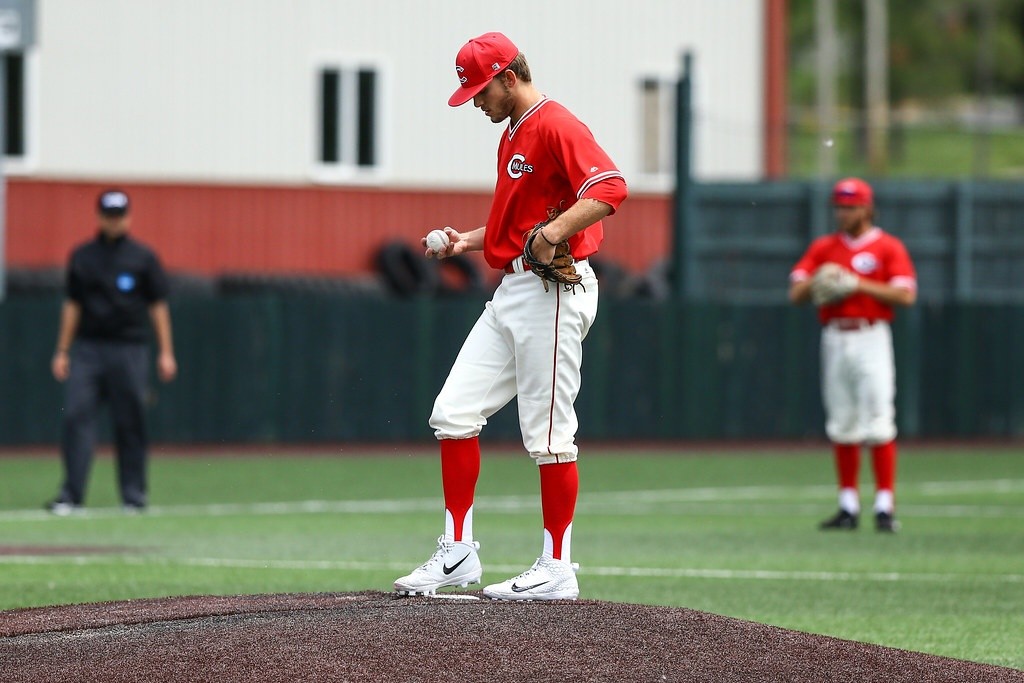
[43,498,81,514]
[876,512,894,532]
[127,500,157,514]
[822,509,858,529]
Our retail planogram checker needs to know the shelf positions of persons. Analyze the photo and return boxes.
[49,187,177,514]
[786,178,918,532]
[394,30,629,602]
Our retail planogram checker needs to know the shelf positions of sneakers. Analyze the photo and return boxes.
[394,534,482,596]
[483,556,579,601]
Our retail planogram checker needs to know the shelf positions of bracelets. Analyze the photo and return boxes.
[540,230,559,246]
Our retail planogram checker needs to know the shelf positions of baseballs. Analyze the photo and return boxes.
[426,230,451,253]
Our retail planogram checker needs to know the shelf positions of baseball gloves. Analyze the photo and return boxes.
[811,262,856,307]
[523,220,583,287]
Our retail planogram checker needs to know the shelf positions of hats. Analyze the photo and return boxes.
[448,32,518,106]
[96,190,128,215]
[834,177,873,207]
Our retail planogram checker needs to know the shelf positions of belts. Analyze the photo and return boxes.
[505,258,532,273]
[838,317,876,330]
